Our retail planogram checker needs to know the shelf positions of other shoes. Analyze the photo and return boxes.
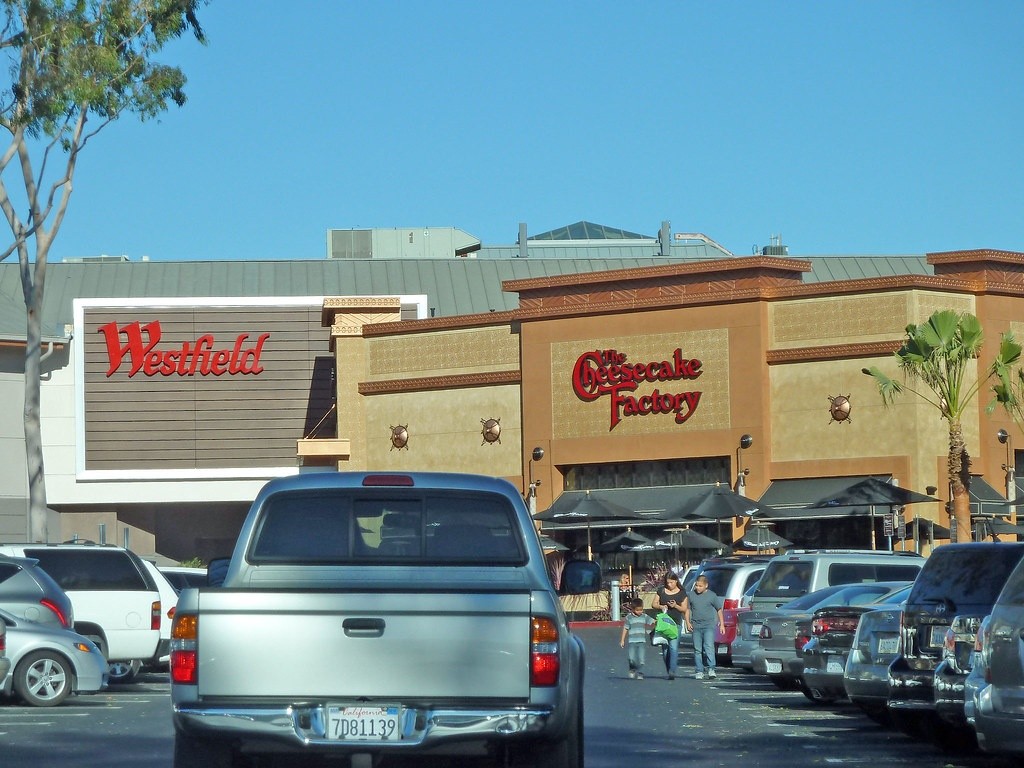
[629,669,636,679]
[668,672,674,680]
[637,671,644,680]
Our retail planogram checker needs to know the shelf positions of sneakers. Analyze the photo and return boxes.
[695,671,704,680]
[708,667,717,679]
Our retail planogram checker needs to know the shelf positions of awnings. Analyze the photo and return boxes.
[541,482,732,529]
[968,476,1010,515]
[751,475,893,520]
[1015,477,1024,516]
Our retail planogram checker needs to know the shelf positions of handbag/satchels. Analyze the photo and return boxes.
[650,609,669,646]
[655,612,678,640]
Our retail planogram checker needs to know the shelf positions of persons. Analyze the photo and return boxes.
[619,574,630,591]
[685,575,725,680]
[651,571,688,679]
[620,598,657,679]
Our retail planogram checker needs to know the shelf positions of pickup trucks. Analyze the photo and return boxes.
[166,470,587,768]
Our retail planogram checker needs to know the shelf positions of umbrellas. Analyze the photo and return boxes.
[886,515,950,555]
[572,531,613,554]
[652,482,785,542]
[802,474,943,550]
[596,527,670,585]
[538,530,572,550]
[971,513,1024,534]
[627,525,729,562]
[723,521,794,556]
[531,490,650,560]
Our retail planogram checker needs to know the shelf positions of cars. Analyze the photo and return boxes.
[802,584,920,702]
[747,584,863,676]
[0,537,209,684]
[0,607,113,709]
[677,554,777,655]
[731,549,932,677]
[842,598,962,738]
[0,553,75,634]
[889,540,1022,768]
